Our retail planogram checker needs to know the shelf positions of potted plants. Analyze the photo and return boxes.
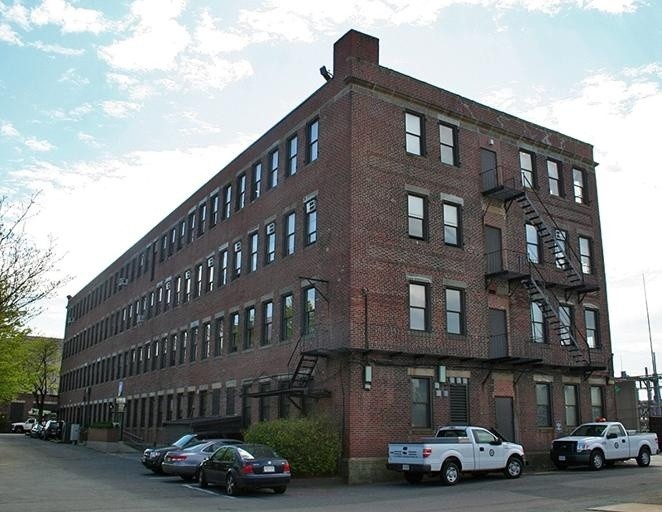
[86,421,119,442]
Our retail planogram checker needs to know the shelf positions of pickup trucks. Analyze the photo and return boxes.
[550,417,660,470]
[386,424,526,485]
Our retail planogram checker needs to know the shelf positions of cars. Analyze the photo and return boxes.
[198,443,291,496]
[141,431,244,473]
[162,438,243,482]
[11,416,66,440]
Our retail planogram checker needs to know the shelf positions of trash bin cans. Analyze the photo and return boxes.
[649,415,662,451]
